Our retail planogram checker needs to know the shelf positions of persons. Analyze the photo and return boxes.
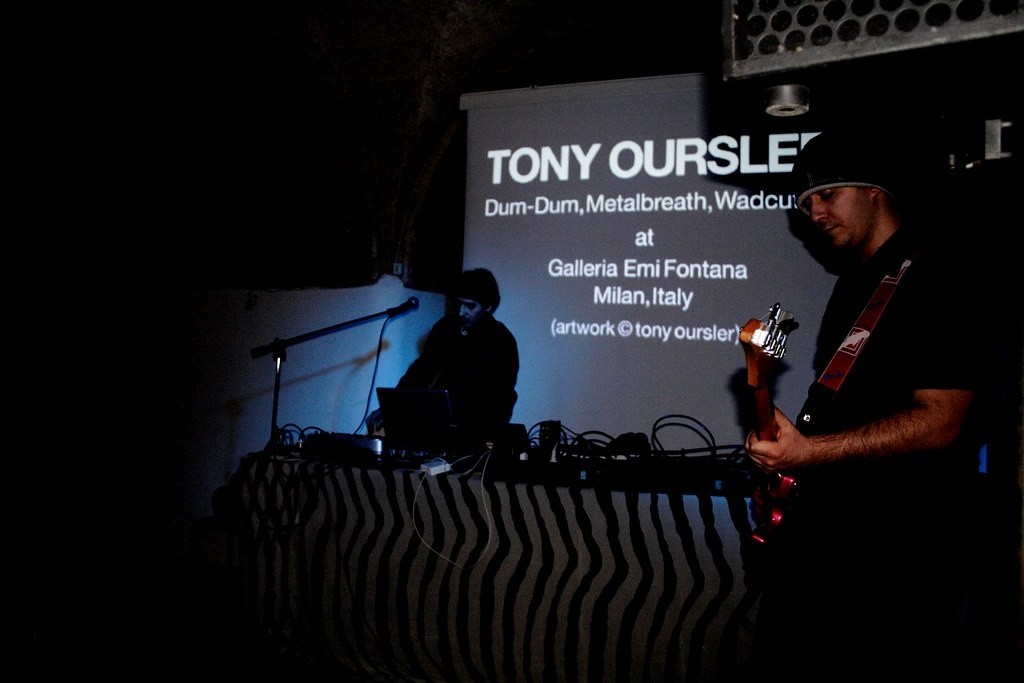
[367,269,519,441]
[743,121,977,682]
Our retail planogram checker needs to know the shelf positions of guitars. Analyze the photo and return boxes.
[740,303,799,543]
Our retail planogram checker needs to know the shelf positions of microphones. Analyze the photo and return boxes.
[391,296,419,318]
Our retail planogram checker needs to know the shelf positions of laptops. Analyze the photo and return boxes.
[377,388,459,457]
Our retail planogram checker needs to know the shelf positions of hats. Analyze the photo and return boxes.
[792,127,896,216]
[449,268,500,307]
